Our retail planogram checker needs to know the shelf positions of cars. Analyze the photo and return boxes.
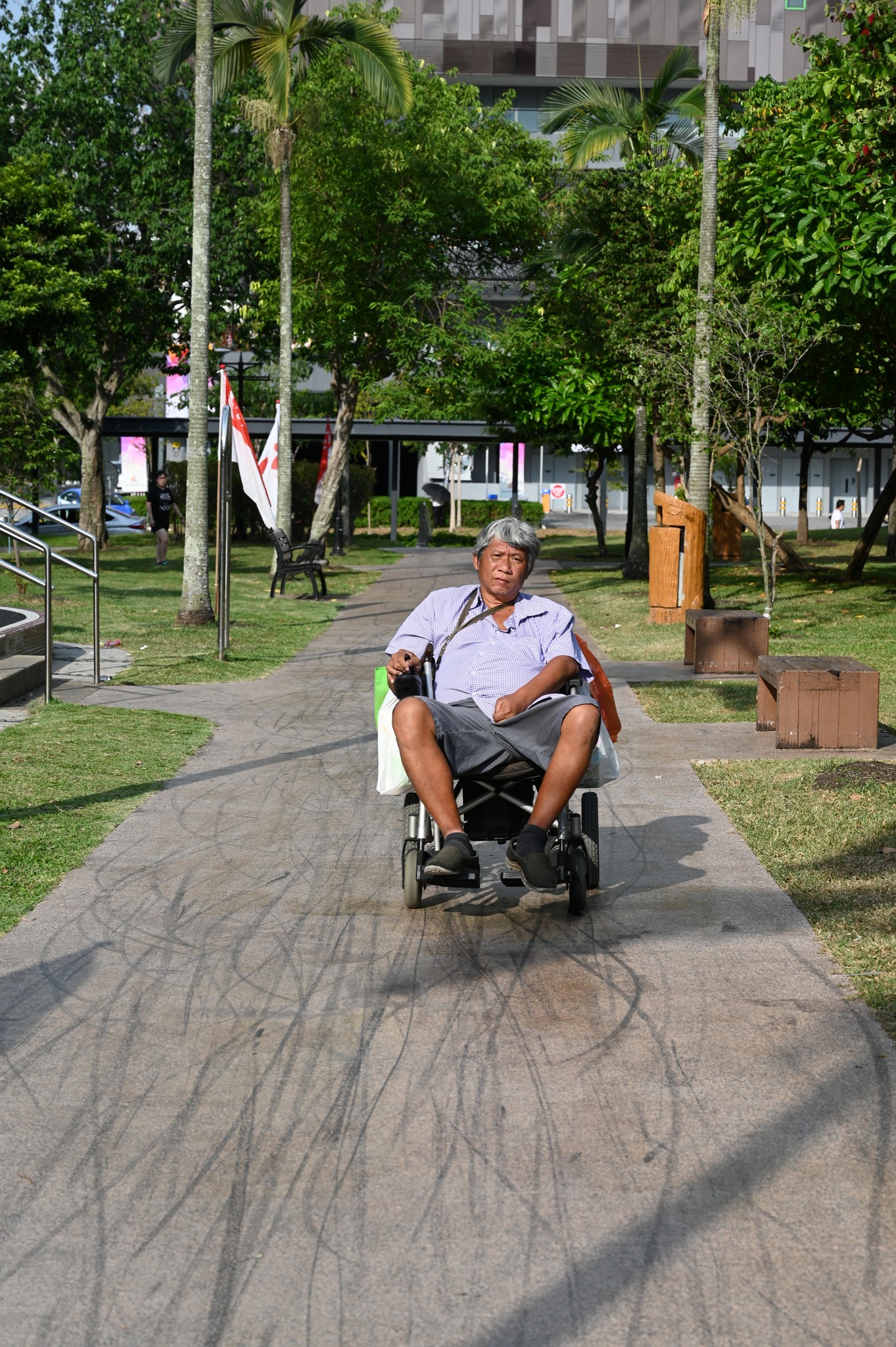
[3,504,148,538]
[57,488,136,518]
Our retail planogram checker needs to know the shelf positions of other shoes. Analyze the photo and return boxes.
[424,840,481,875]
[505,837,559,892]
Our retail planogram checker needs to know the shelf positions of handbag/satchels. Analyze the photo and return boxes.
[575,717,620,789]
[377,687,416,796]
[572,633,621,743]
[374,666,391,726]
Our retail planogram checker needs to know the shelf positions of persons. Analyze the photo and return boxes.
[830,500,845,530]
[430,495,447,528]
[147,471,185,565]
[385,517,599,892]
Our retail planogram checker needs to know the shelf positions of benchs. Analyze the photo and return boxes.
[757,655,880,750]
[683,609,770,674]
[267,527,329,601]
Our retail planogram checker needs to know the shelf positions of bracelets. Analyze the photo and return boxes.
[179,517,183,520]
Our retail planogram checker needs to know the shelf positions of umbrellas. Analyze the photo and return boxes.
[421,483,451,506]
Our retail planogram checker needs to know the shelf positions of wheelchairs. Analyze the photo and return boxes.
[391,642,601,917]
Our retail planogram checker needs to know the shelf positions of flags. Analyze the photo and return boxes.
[257,409,279,524]
[315,422,332,505]
[220,368,277,529]
